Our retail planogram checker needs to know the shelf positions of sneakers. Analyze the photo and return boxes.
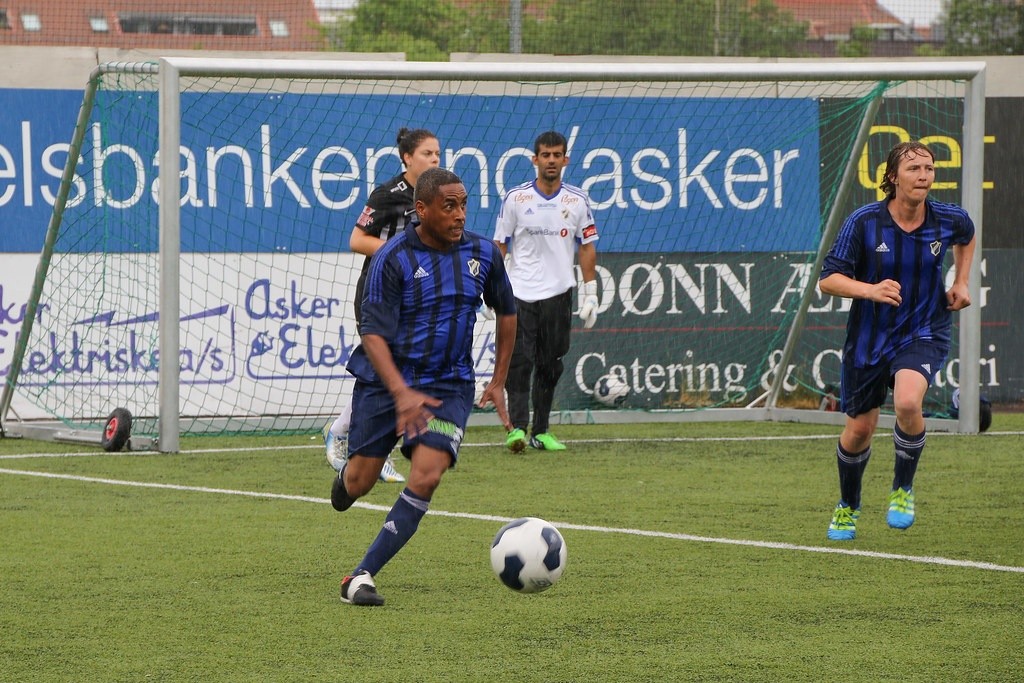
[321,421,349,470]
[339,569,384,606]
[505,427,527,452]
[886,486,915,529]
[828,498,860,541]
[378,461,406,482]
[530,433,568,451]
[332,463,358,511]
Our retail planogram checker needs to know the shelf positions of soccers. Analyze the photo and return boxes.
[593,373,630,408]
[490,518,567,594]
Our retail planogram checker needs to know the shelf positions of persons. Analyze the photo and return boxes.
[331,166,517,607]
[481,130,601,455]
[818,141,976,541]
[321,126,443,485]
